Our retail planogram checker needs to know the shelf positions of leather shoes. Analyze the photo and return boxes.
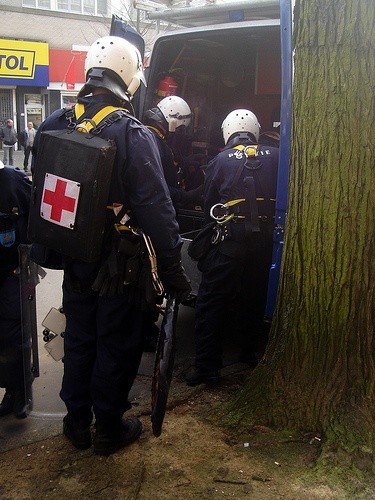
[15,385,33,418]
[62,413,92,448]
[0,393,14,416]
[93,418,142,455]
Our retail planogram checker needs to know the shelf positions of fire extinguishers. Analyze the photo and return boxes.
[155,67,182,106]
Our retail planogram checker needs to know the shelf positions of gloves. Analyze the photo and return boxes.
[155,243,193,306]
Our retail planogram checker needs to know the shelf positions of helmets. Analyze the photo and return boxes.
[221,109,261,145]
[157,95,191,133]
[84,36,148,98]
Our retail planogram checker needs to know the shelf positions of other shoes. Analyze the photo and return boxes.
[238,352,258,367]
[184,366,219,385]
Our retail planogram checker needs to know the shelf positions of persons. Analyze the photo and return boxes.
[186,109,279,387]
[23,122,37,170]
[0,119,17,166]
[31,36,192,453]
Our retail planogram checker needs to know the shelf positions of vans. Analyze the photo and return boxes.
[139,0,293,328]
